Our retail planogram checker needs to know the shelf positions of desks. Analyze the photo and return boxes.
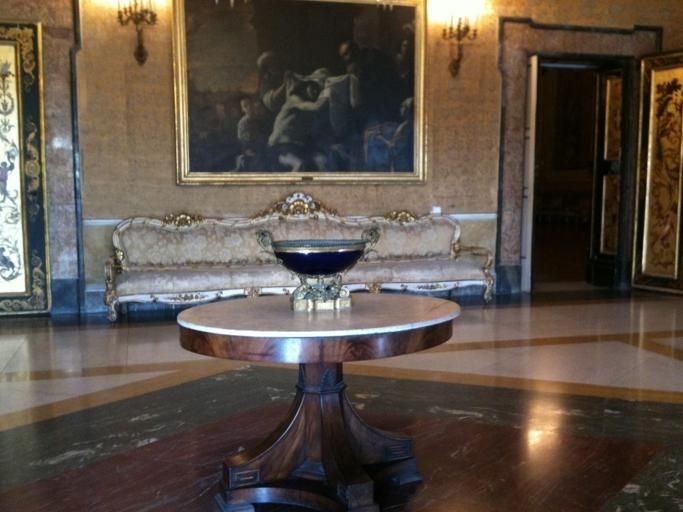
[177,295,459,511]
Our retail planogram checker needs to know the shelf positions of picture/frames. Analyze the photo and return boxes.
[171,0,429,187]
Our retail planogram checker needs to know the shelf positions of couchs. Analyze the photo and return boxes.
[103,191,494,322]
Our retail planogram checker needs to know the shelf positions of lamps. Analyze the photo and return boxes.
[442,13,479,77]
[116,0,157,63]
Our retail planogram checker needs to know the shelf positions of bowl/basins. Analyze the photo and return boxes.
[255,229,382,313]
